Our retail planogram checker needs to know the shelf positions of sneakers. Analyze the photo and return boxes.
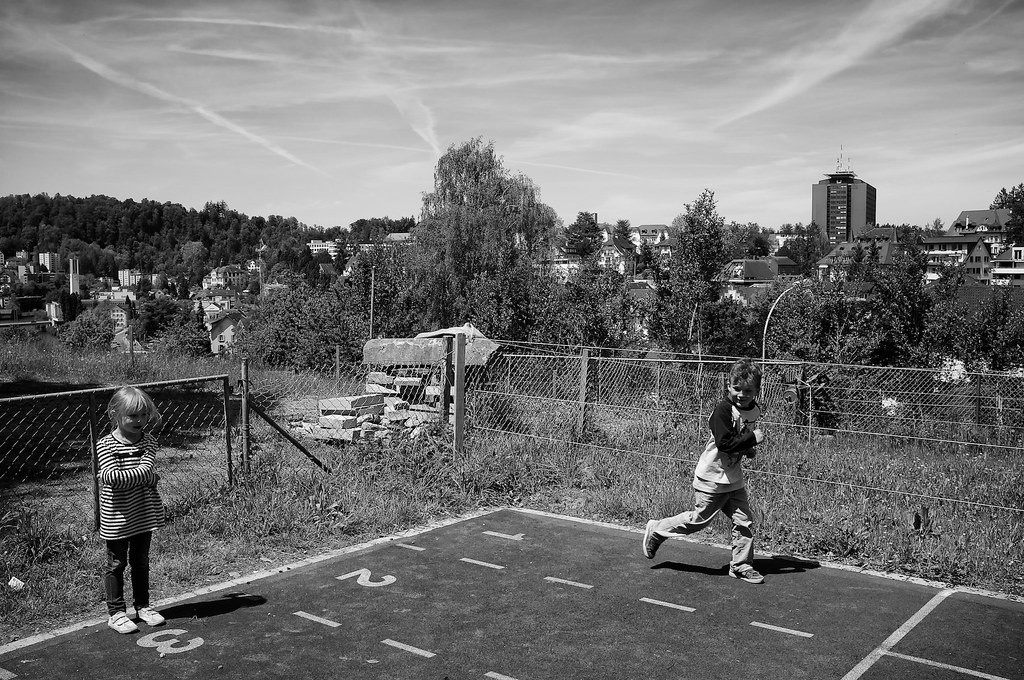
[136,608,165,625]
[107,612,138,634]
[643,520,667,559]
[729,566,764,583]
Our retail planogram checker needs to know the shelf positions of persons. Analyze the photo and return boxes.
[96,386,166,634]
[642,358,765,584]
